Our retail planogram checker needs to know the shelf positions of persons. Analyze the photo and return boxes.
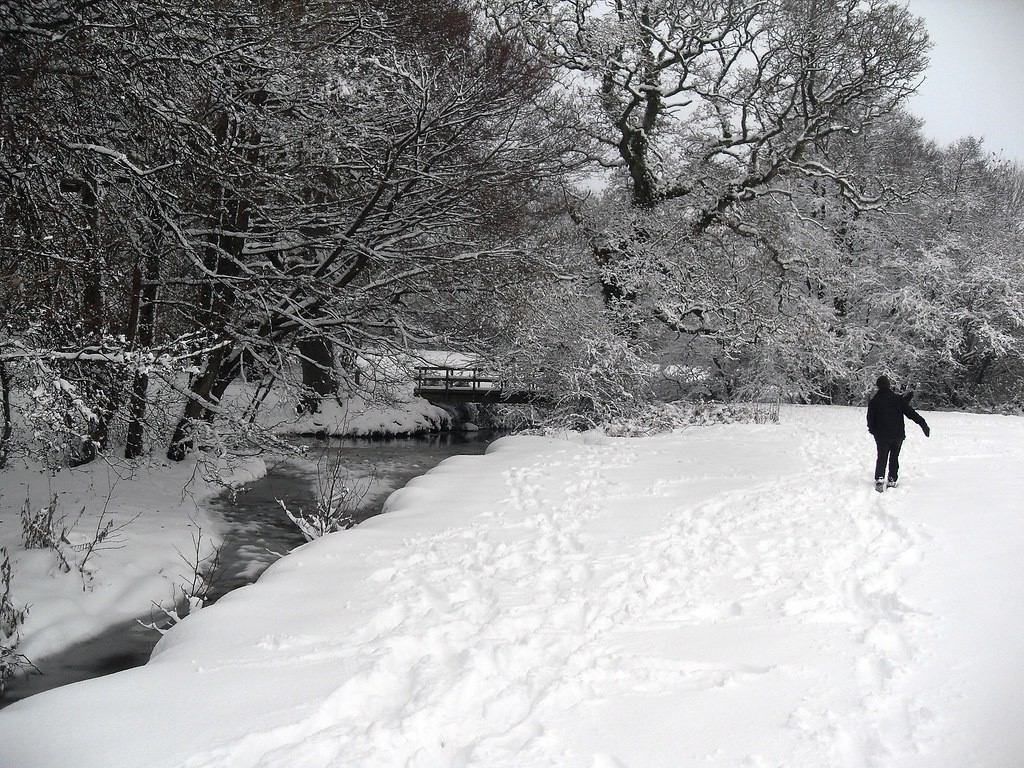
[866,376,930,493]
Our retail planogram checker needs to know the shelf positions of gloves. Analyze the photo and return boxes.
[923,425,930,437]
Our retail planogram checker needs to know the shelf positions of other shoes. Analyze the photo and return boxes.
[876,483,883,492]
[886,481,896,488]
